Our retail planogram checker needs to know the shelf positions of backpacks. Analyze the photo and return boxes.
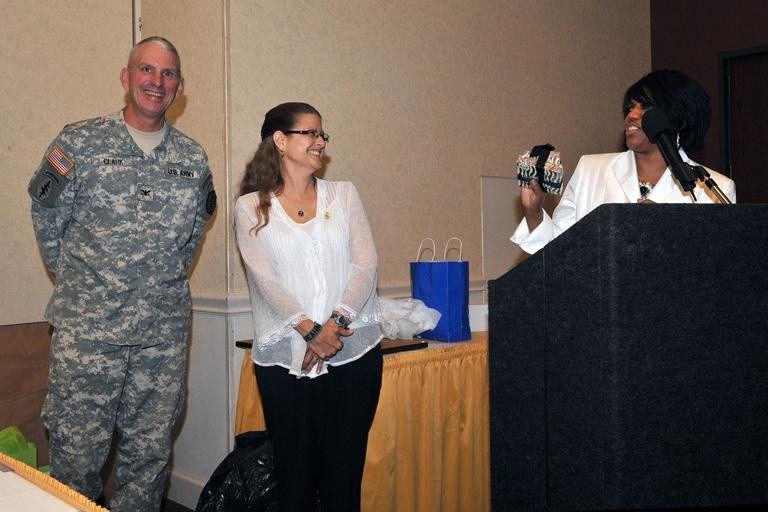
[194,427,281,512]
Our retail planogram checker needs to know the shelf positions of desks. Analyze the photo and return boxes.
[235,331,491,511]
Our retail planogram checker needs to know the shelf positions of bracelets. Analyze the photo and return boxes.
[304,323,321,342]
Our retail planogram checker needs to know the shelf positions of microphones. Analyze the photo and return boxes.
[641,106,699,192]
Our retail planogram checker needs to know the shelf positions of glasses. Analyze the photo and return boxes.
[284,129,330,142]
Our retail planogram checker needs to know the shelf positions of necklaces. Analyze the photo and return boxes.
[278,190,317,217]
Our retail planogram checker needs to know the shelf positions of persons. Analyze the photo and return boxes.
[236,100,382,512]
[30,35,216,512]
[510,67,735,259]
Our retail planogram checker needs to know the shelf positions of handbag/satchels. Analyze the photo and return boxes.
[406,236,476,344]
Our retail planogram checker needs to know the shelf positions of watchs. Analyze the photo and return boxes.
[329,314,346,328]
